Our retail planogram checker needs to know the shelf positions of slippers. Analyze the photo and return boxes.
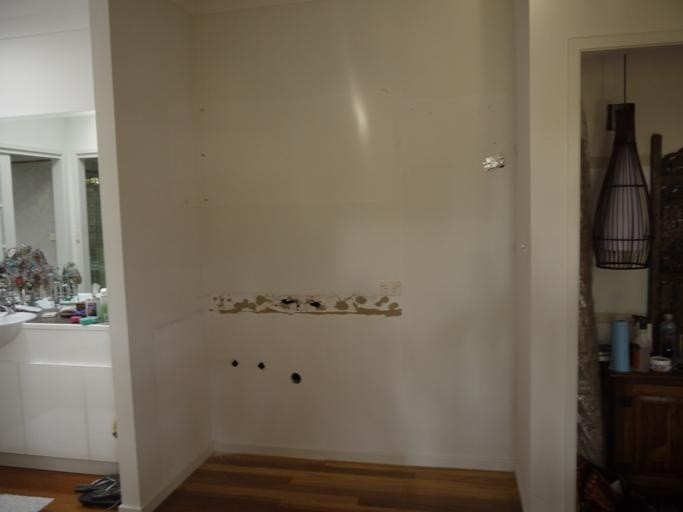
[76,473,120,507]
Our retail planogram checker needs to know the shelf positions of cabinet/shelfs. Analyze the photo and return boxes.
[0,358,118,475]
[599,359,683,506]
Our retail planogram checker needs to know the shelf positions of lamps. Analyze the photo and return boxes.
[591,53,653,270]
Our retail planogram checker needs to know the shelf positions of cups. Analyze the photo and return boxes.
[610,319,631,373]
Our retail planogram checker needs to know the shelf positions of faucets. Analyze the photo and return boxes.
[54,279,69,305]
[2,296,16,314]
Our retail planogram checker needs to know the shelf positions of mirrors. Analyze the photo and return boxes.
[0,110,107,329]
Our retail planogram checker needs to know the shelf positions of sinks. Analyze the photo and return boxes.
[0,311,37,345]
[35,293,94,310]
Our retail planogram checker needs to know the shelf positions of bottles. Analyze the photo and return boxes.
[660,314,676,361]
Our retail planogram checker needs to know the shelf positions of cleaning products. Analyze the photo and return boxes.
[630,314,651,371]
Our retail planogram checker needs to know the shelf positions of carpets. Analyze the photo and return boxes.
[0,493,55,512]
